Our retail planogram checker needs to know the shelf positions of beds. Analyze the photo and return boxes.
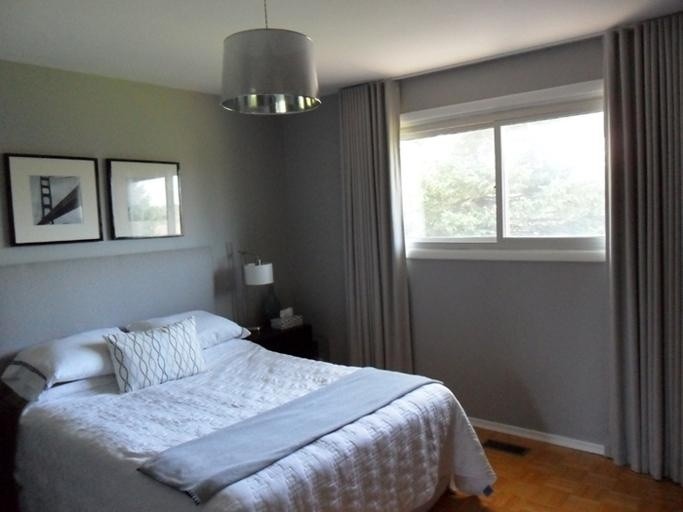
[0,309,497,511]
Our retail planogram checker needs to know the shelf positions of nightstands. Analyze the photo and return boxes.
[243,324,312,358]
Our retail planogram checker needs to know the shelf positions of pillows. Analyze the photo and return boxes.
[126,310,252,352]
[101,316,209,395]
[0,326,126,402]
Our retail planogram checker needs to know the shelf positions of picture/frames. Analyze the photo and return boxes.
[4,153,104,247]
[106,159,184,240]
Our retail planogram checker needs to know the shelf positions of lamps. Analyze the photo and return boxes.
[240,250,275,287]
[218,0,323,116]
